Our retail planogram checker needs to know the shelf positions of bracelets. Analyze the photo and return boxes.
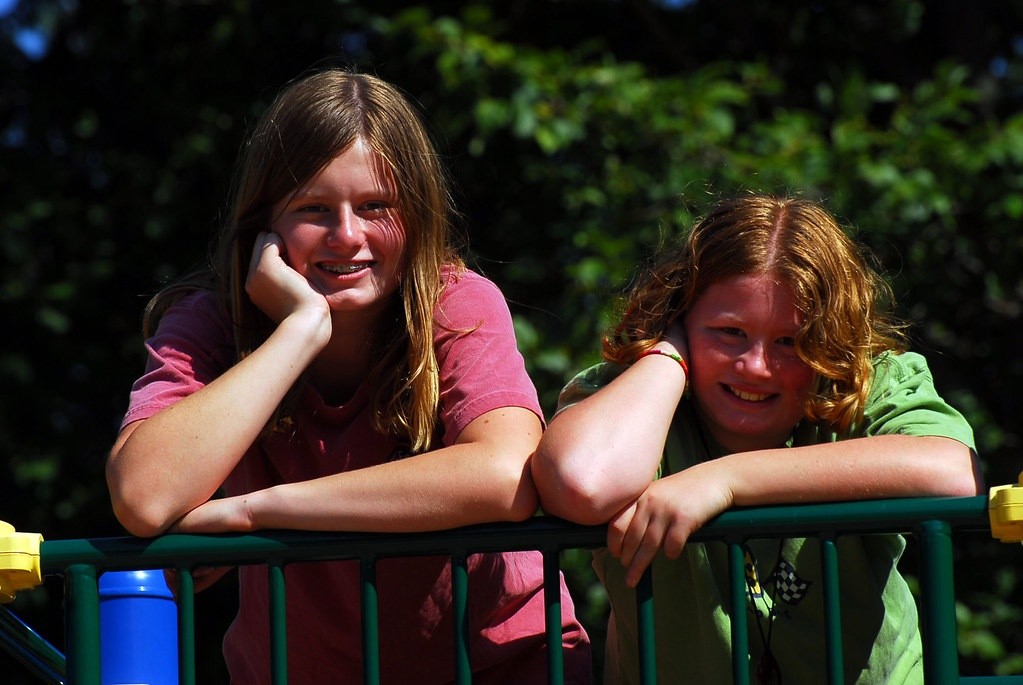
[632,349,690,395]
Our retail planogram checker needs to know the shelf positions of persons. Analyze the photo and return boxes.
[533,196,976,685]
[103,72,599,685]
